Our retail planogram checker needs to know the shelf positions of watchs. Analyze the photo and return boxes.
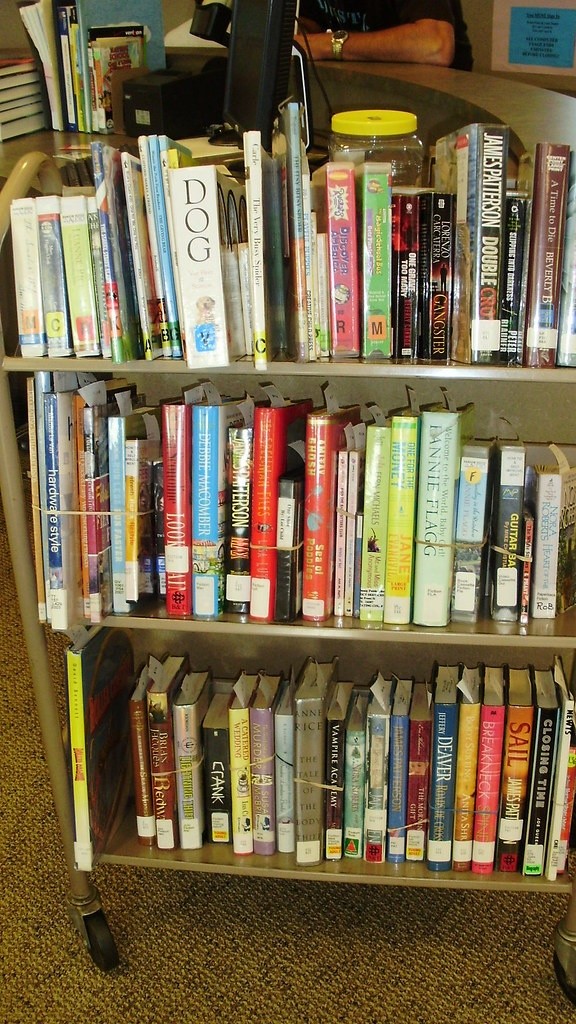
[331,30,349,61]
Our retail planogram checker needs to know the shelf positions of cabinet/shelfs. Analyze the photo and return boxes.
[0,150,576,1006]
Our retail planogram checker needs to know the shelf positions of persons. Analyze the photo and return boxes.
[292,0,476,71]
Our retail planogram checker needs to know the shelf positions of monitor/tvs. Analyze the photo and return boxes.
[223,0,329,178]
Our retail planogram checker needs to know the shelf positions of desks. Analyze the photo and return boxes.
[0,47,576,198]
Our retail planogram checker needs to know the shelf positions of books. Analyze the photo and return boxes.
[8,102,576,370]
[65,626,576,881]
[0,47,48,141]
[20,0,167,135]
[26,370,576,629]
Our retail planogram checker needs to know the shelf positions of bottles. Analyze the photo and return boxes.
[327,108,423,191]
